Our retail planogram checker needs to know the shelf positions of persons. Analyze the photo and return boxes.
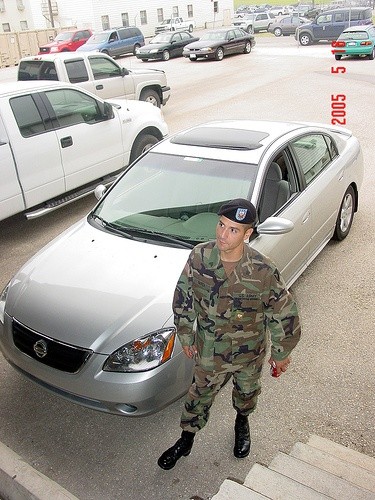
[158,199,301,470]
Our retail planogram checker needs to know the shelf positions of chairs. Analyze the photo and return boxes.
[258,162,292,224]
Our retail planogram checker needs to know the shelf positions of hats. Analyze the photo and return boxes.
[216,198,257,225]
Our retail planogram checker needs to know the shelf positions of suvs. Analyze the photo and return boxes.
[76,26,145,57]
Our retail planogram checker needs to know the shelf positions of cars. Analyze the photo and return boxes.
[267,15,313,38]
[1,117,364,417]
[38,29,94,55]
[236,4,324,17]
[333,25,375,61]
[239,12,279,34]
[136,31,200,62]
[182,26,256,62]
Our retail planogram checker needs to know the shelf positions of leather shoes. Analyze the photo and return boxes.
[233,413,252,458]
[156,429,195,471]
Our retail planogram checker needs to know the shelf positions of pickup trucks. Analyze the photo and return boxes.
[154,17,196,34]
[15,50,170,109]
[2,81,170,226]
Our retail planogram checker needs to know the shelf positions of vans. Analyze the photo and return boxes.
[294,7,373,47]
